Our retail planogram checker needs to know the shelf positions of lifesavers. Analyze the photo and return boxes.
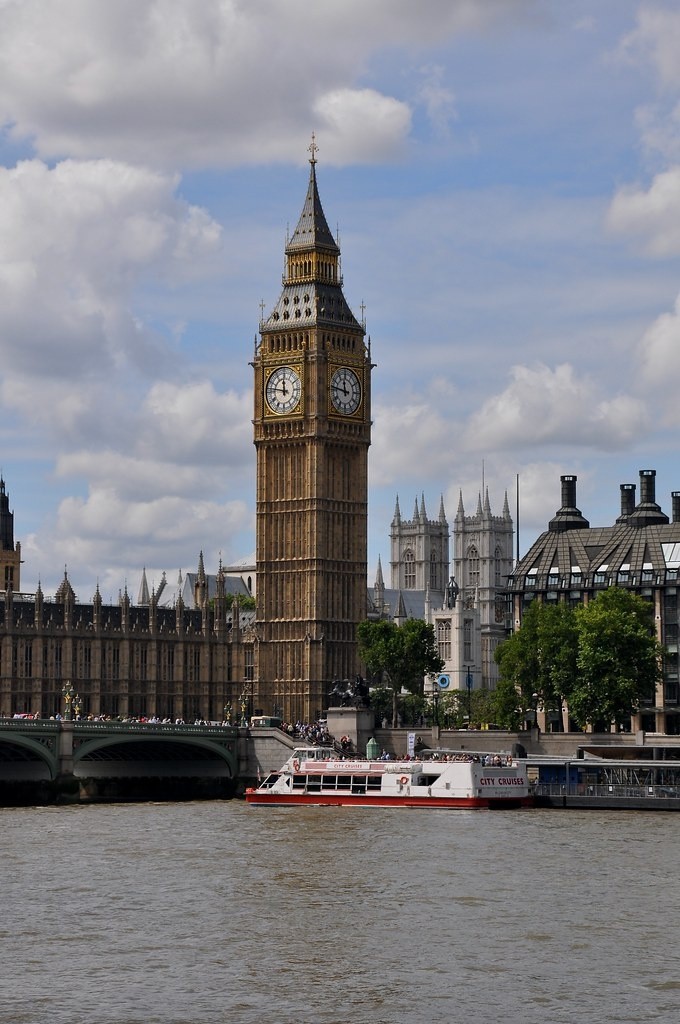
[400,776,408,784]
[295,764,300,771]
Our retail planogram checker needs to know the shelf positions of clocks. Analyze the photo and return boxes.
[263,366,302,415]
[330,366,363,417]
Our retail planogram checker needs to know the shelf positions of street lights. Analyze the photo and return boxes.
[62,681,74,719]
[72,693,83,720]
[462,663,476,725]
[224,702,232,725]
[532,692,540,729]
[434,687,439,726]
[237,694,248,726]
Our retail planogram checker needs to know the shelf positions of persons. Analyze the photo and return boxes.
[13,712,40,719]
[50,712,65,720]
[535,777,538,786]
[222,719,260,727]
[76,713,211,731]
[313,748,512,767]
[283,720,335,742]
[341,735,351,748]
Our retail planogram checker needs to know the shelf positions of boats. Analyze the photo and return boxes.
[243,745,538,810]
[528,747,680,810]
